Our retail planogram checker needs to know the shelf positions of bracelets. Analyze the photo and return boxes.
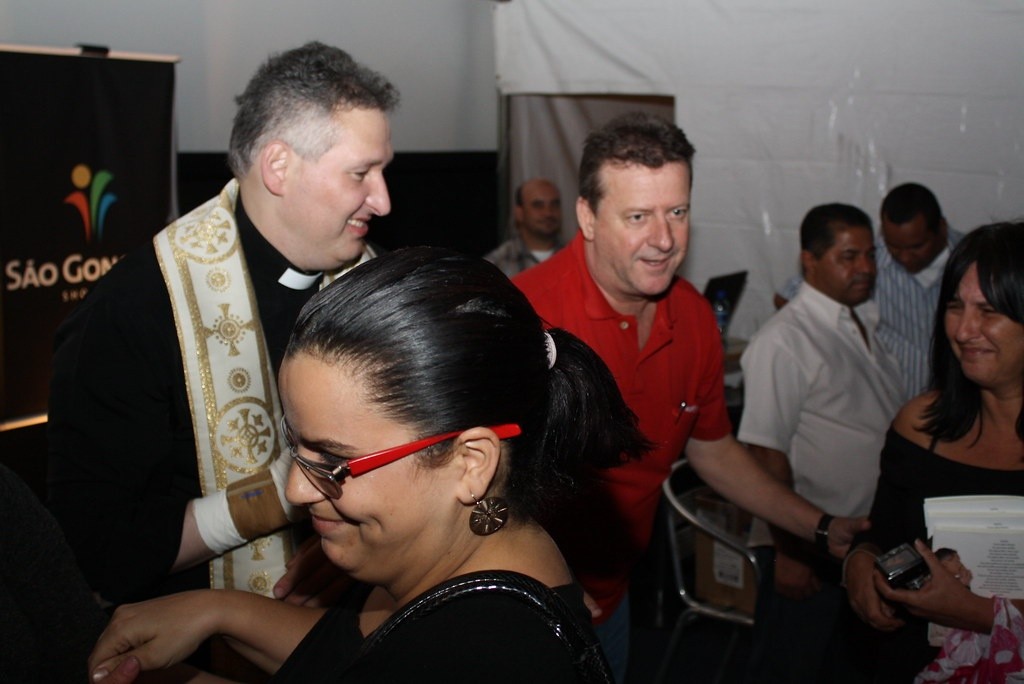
[815,513,833,554]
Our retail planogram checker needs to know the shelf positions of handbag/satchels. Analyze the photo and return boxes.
[914,592,1024,684]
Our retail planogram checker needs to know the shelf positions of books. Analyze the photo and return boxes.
[921,494,1024,646]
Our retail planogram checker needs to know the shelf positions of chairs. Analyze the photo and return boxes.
[654,459,768,684]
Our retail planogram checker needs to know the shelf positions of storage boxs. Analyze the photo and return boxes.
[691,487,769,617]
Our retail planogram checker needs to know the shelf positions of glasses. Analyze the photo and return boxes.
[280,414,523,498]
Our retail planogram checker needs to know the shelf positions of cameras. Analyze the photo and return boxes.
[873,543,930,591]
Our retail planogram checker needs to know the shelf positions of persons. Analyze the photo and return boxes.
[485,179,567,279]
[928,546,972,646]
[511,111,874,684]
[89,239,660,684]
[875,539,1024,683]
[47,36,401,611]
[771,182,972,401]
[873,221,1024,684]
[736,201,908,684]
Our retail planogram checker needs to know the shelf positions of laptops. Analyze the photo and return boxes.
[702,269,748,323]
